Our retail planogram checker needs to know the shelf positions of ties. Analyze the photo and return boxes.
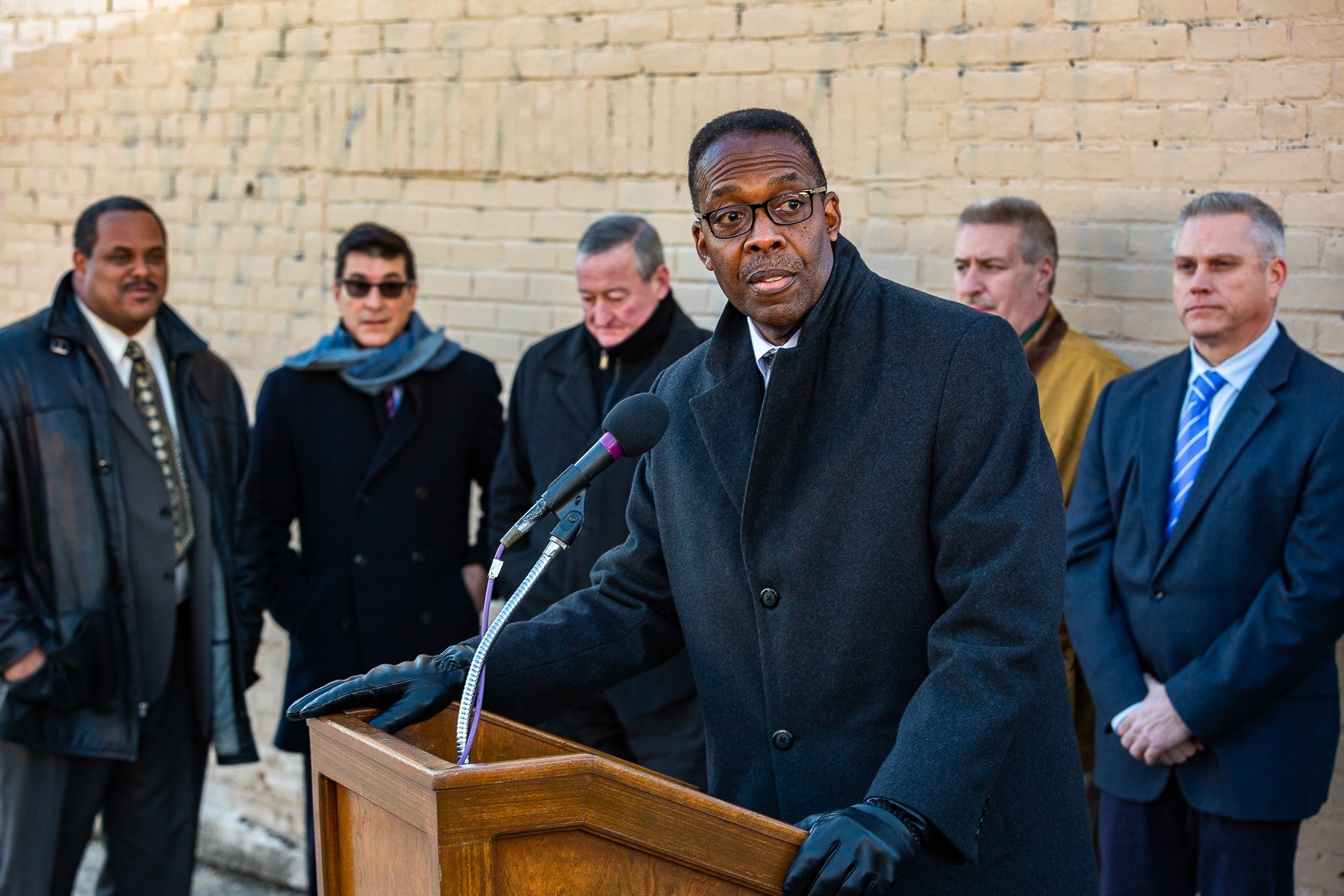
[1162,369,1226,540]
[124,343,195,567]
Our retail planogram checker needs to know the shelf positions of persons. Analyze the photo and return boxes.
[0,196,264,896]
[484,215,714,789]
[1063,196,1344,896]
[233,222,507,896]
[288,108,1103,896]
[952,197,1135,842]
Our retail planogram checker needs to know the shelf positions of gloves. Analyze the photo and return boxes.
[286,642,472,736]
[784,799,925,896]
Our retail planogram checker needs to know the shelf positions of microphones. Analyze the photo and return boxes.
[500,392,671,549]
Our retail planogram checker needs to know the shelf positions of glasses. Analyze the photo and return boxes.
[695,186,826,239]
[339,278,410,297]
